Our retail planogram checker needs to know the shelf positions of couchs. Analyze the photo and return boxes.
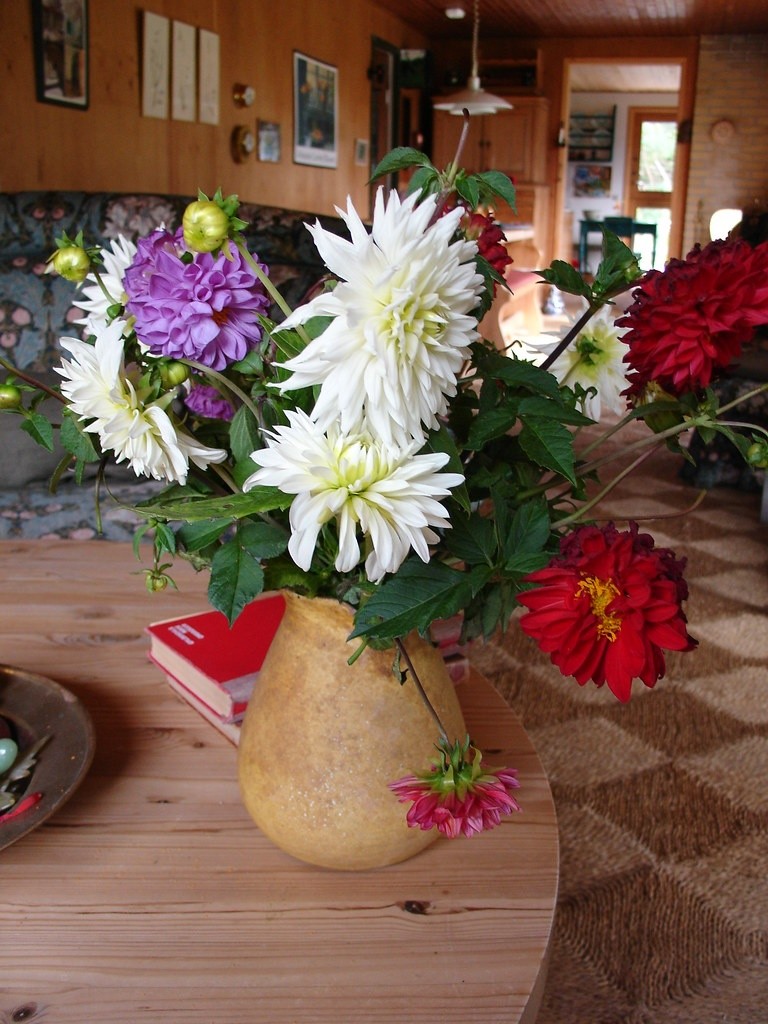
[0,189,372,543]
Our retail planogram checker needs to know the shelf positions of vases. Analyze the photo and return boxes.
[238,585,465,875]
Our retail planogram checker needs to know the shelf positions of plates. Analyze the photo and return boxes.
[0,664,94,851]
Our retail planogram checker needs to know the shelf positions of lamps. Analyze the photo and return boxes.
[431,0,515,119]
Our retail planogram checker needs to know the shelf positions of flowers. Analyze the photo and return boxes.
[42,145,768,840]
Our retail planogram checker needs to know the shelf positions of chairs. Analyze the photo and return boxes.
[602,216,642,264]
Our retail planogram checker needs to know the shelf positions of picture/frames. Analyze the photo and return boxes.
[30,0,92,108]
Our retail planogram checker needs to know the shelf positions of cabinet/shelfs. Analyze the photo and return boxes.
[568,101,617,164]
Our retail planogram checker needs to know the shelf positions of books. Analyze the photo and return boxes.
[146,586,486,749]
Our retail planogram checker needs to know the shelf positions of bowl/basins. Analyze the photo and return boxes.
[583,211,602,220]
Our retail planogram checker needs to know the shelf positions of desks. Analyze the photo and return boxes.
[579,220,658,281]
[0,539,565,1024]
